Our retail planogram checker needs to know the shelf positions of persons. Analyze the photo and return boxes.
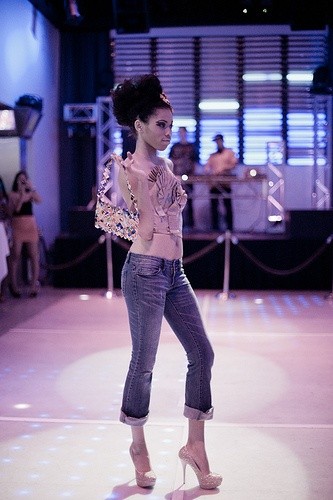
[168,127,197,230]
[0,170,43,302]
[110,74,223,490]
[204,134,236,233]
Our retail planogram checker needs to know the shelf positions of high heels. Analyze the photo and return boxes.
[177,447,223,492]
[130,444,156,488]
